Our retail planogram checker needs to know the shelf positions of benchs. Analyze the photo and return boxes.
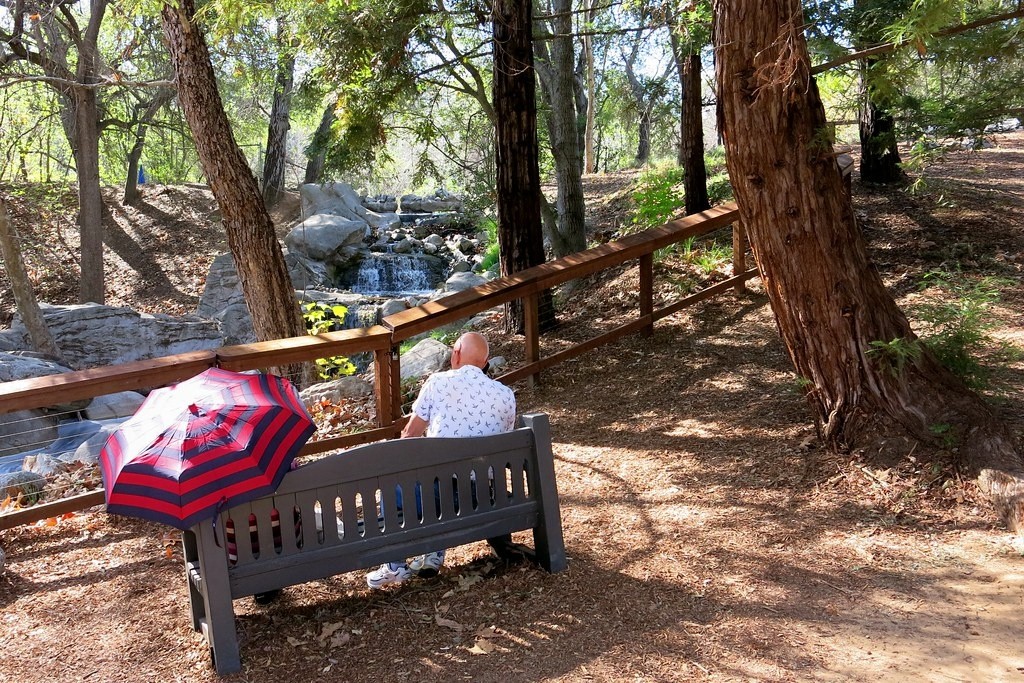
[180,412,568,677]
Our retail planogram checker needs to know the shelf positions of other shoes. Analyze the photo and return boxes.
[254,589,275,607]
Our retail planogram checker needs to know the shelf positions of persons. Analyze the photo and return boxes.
[367,332,516,589]
[227,459,303,607]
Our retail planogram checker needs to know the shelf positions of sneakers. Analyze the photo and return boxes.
[366,561,412,588]
[409,551,444,578]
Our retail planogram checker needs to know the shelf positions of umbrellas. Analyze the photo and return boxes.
[98,367,318,530]
